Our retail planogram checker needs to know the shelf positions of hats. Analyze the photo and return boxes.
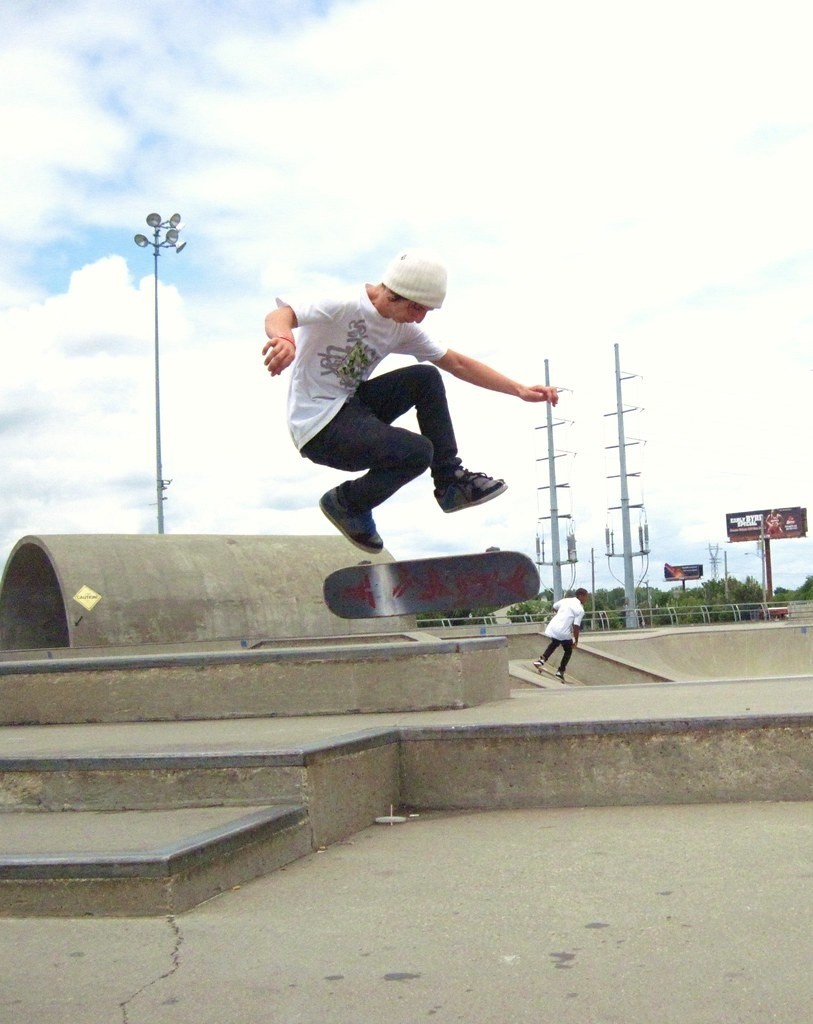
[381,250,447,309]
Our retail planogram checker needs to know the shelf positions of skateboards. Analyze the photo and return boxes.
[532,662,575,686]
[322,547,542,621]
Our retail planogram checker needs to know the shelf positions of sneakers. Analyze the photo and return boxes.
[555,672,565,684]
[533,660,544,669]
[319,487,384,554]
[433,469,508,513]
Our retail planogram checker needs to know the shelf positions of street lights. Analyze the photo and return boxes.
[134,213,187,534]
[745,552,766,603]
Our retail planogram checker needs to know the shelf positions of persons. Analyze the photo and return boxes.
[262,249,558,555]
[534,588,588,680]
[766,509,784,534]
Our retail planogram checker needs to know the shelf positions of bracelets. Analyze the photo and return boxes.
[278,335,297,350]
